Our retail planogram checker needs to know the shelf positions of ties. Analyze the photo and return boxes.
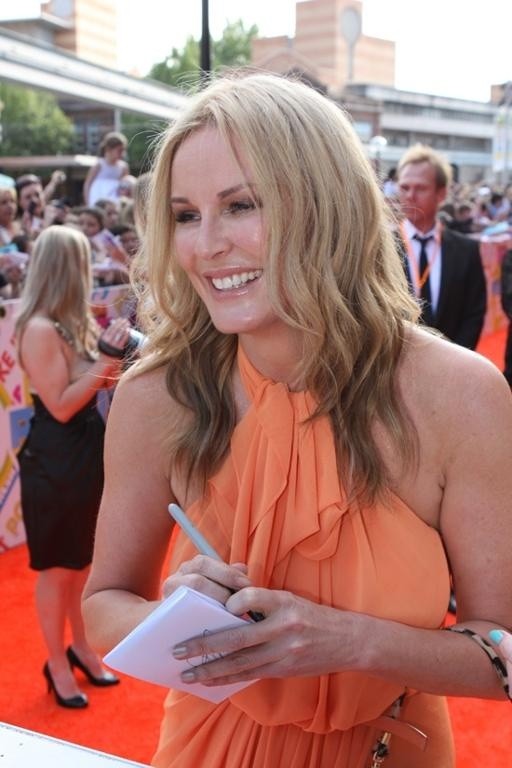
[414,236,432,325]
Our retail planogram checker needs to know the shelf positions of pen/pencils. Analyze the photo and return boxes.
[168,502,265,622]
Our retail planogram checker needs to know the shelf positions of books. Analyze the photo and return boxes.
[100,584,272,707]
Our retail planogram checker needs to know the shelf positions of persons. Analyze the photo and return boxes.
[496,249,512,390]
[10,222,131,708]
[0,123,145,301]
[76,63,511,766]
[486,625,512,690]
[370,156,512,240]
[390,141,489,354]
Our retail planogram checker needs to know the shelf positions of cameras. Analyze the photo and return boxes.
[97,318,151,355]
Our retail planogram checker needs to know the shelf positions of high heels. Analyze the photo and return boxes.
[43,646,120,707]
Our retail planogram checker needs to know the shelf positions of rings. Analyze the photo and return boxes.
[115,332,122,343]
